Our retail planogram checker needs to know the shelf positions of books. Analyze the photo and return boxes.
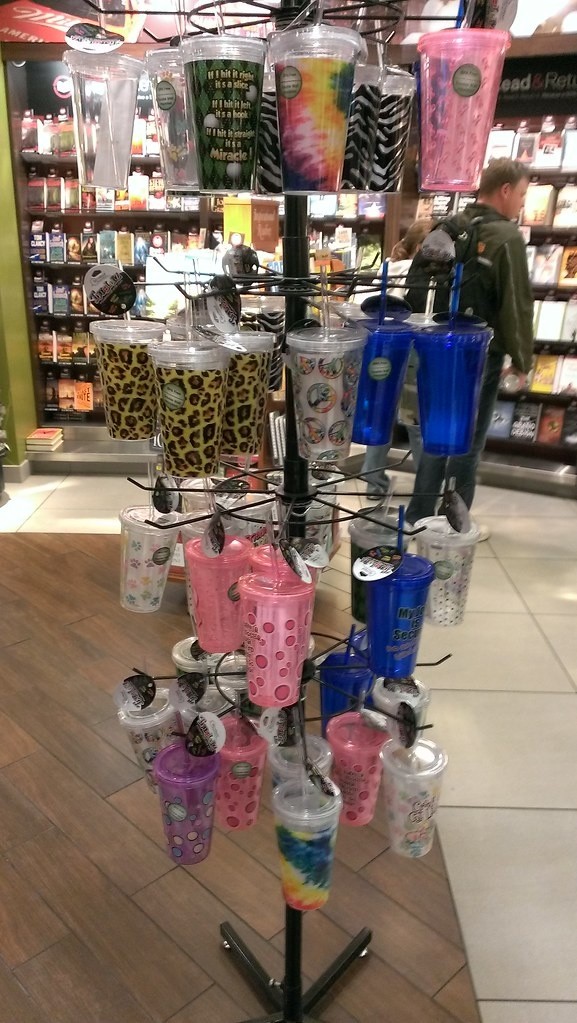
[26,435,65,452]
[26,428,63,445]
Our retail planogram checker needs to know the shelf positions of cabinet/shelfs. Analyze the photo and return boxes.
[0,33,577,466]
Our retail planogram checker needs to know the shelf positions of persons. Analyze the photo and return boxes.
[396,157,535,547]
[360,218,447,502]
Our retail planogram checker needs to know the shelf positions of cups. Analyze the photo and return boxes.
[271,777,344,911]
[62,49,144,191]
[319,651,371,739]
[117,687,182,795]
[268,731,334,789]
[417,28,510,191]
[89,312,276,481]
[352,629,369,658]
[118,470,340,652]
[373,677,430,740]
[348,510,480,680]
[380,735,448,857]
[215,715,268,830]
[177,683,240,743]
[146,25,415,194]
[172,625,315,716]
[154,741,221,866]
[236,570,316,708]
[326,711,389,828]
[287,299,497,463]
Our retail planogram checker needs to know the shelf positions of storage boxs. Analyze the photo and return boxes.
[221,194,279,254]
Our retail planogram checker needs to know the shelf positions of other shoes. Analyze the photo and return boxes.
[475,524,491,543]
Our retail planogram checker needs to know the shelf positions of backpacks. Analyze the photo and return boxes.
[402,213,510,313]
